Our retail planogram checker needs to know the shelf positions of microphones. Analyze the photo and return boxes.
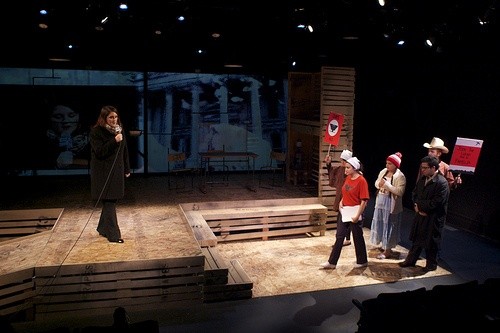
[115,127,120,134]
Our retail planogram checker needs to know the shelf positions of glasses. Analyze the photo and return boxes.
[419,166,431,170]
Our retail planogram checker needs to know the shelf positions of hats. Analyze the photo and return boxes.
[346,157,360,170]
[340,149,352,160]
[386,152,402,167]
[423,137,448,154]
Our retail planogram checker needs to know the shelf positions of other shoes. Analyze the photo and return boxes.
[96,226,123,243]
[354,262,368,268]
[421,265,437,271]
[320,262,335,268]
[332,241,351,247]
[376,250,392,259]
[399,258,415,267]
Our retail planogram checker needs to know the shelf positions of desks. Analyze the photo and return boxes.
[198,152,256,194]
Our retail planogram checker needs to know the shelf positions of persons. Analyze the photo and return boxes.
[369,151,406,260]
[319,157,368,268]
[15,99,91,170]
[89,106,131,243]
[324,149,357,247]
[410,136,462,263]
[398,156,449,271]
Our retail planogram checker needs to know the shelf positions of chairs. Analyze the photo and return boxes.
[258,148,287,190]
[168,147,193,190]
